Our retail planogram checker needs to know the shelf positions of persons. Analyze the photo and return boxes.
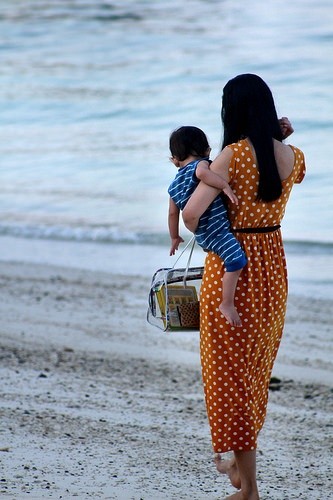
[167,124,248,327]
[182,72,307,500]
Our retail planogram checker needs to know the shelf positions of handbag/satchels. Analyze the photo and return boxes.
[146,236,204,332]
